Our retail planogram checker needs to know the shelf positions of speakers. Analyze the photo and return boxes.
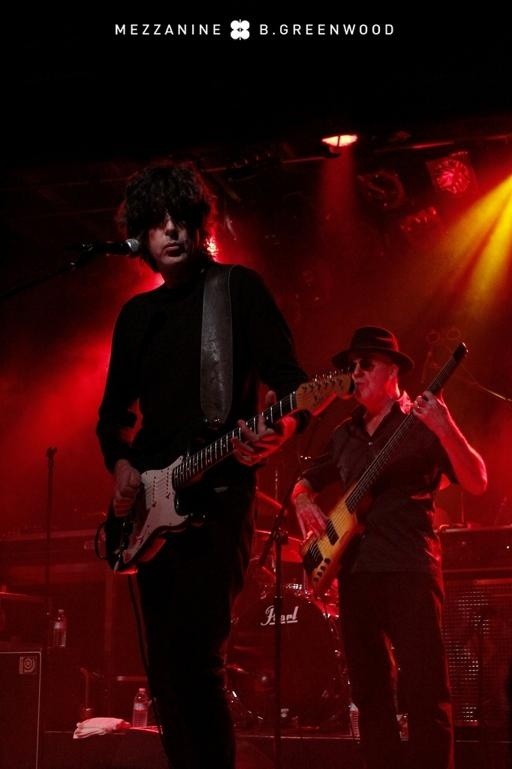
[42,574,136,712]
[437,577,512,727]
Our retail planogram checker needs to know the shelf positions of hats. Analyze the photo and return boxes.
[330,326,414,374]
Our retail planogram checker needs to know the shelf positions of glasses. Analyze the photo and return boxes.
[343,357,394,372]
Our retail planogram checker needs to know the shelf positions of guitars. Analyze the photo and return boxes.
[103,367,355,574]
[298,341,467,596]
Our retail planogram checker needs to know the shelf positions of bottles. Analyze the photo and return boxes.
[132,688,148,727]
[53,609,67,647]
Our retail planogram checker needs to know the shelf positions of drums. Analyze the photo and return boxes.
[229,583,397,724]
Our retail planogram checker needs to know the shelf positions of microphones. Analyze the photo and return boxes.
[226,663,270,685]
[94,238,140,256]
[340,377,356,400]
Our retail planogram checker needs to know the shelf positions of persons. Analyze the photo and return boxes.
[95,161,313,768]
[285,319,493,769]
